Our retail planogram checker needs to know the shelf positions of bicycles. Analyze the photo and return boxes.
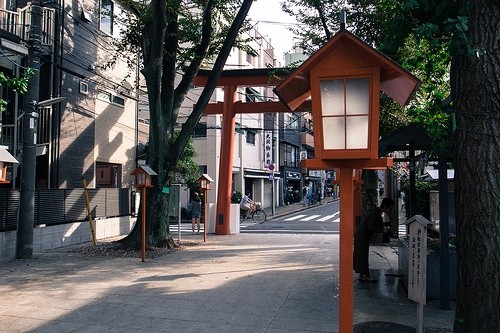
[239,202,266,224]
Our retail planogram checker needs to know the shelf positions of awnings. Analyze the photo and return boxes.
[378,122,427,158]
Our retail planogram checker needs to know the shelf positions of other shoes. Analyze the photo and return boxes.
[197,232,200,235]
[191,232,195,235]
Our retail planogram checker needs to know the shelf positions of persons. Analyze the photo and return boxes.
[191,192,201,235]
[240,191,253,219]
[302,184,310,207]
[353,197,394,282]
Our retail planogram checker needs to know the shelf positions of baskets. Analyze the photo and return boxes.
[257,205,262,210]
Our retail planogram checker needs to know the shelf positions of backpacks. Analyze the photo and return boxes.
[303,187,307,196]
